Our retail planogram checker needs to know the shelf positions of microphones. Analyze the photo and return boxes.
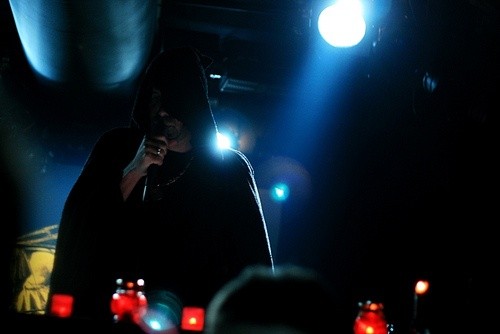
[142,126,168,202]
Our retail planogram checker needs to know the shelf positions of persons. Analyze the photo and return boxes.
[204,264,351,334]
[45,47,274,334]
